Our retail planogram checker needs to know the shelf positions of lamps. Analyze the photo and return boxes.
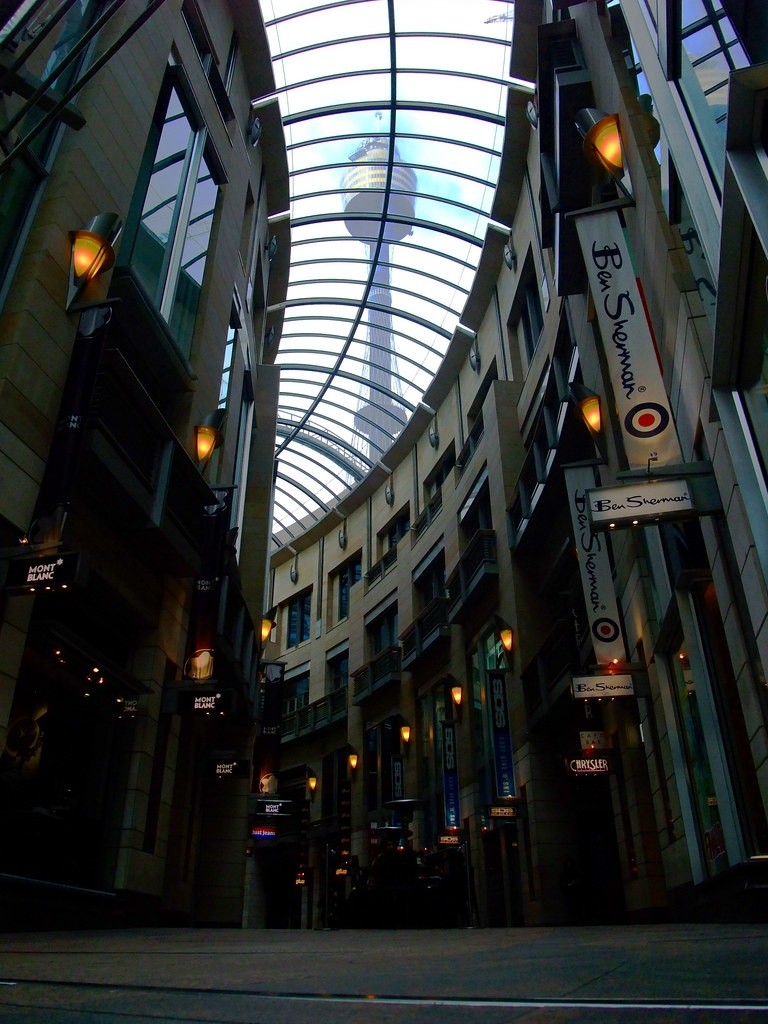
[563,107,636,220]
[65,211,125,311]
[262,605,279,641]
[344,743,358,768]
[446,672,462,705]
[195,408,230,461]
[560,381,606,472]
[307,766,317,790]
[492,612,513,652]
[395,713,410,742]
